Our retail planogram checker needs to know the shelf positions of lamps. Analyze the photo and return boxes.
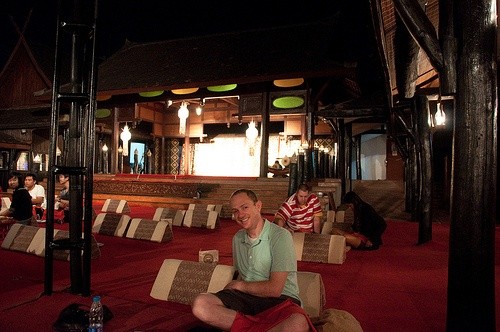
[432,96,447,130]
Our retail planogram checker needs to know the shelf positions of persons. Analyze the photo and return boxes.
[332,192,386,252]
[275,184,323,235]
[54,175,71,223]
[0,171,33,226]
[24,174,46,219]
[191,187,311,332]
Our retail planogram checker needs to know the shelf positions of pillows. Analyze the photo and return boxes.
[0,197,364,321]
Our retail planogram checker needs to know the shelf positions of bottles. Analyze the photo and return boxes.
[89,296,103,332]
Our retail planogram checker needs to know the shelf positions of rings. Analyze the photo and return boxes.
[229,285,231,288]
